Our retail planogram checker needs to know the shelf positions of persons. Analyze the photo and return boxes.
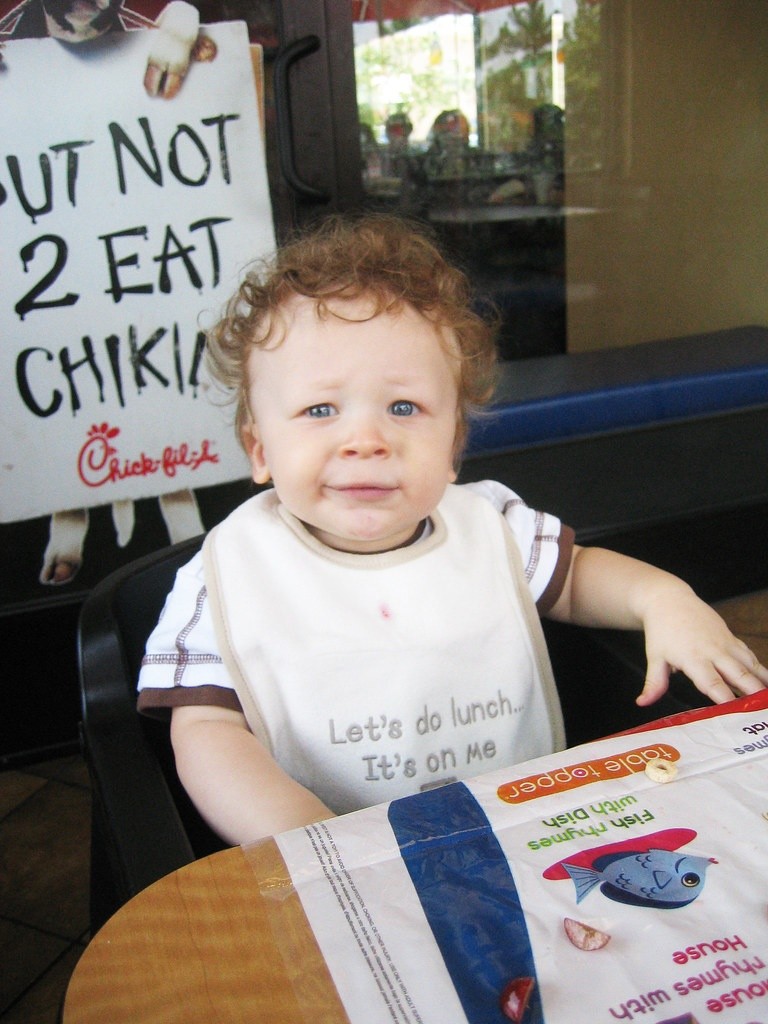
[135,211,768,852]
[377,103,564,189]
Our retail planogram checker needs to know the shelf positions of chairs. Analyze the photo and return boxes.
[73,535,682,932]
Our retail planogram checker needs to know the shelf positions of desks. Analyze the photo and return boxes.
[62,690,768,1024]
[428,198,622,353]
